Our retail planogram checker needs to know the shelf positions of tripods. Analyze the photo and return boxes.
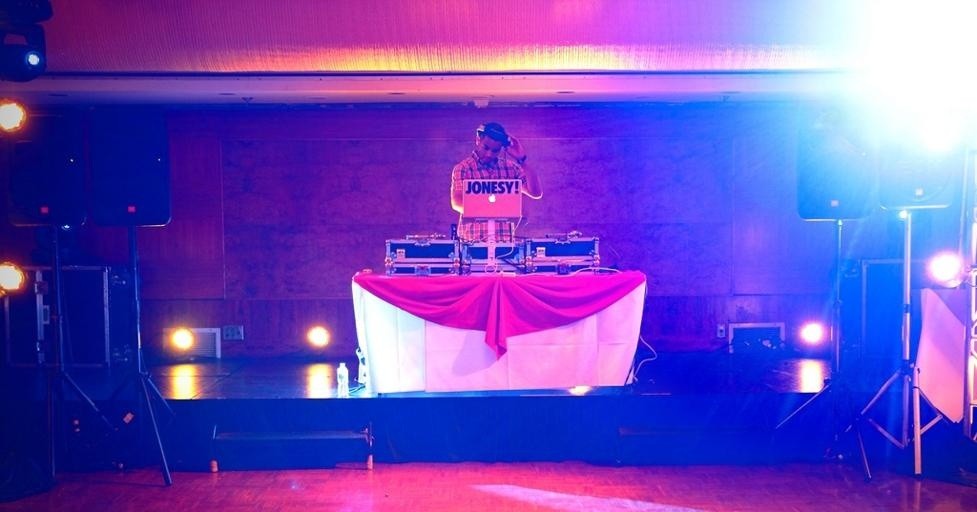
[771,223,883,480]
[88,229,202,487]
[847,214,956,479]
[12,226,111,484]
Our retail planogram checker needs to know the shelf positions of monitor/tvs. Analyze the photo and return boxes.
[462,178,523,218]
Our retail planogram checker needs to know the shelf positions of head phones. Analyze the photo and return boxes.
[478,123,511,149]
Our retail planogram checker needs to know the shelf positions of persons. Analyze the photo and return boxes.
[449,119,543,248]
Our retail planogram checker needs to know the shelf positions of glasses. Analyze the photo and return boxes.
[477,124,510,146]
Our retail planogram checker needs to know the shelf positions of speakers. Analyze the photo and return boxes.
[797,98,874,222]
[877,102,952,211]
[88,104,173,226]
[6,103,81,227]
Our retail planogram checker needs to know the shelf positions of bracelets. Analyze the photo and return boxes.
[518,154,527,164]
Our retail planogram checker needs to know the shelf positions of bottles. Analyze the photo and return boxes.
[336,362,350,399]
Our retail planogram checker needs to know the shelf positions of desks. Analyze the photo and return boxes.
[350,270,649,392]
